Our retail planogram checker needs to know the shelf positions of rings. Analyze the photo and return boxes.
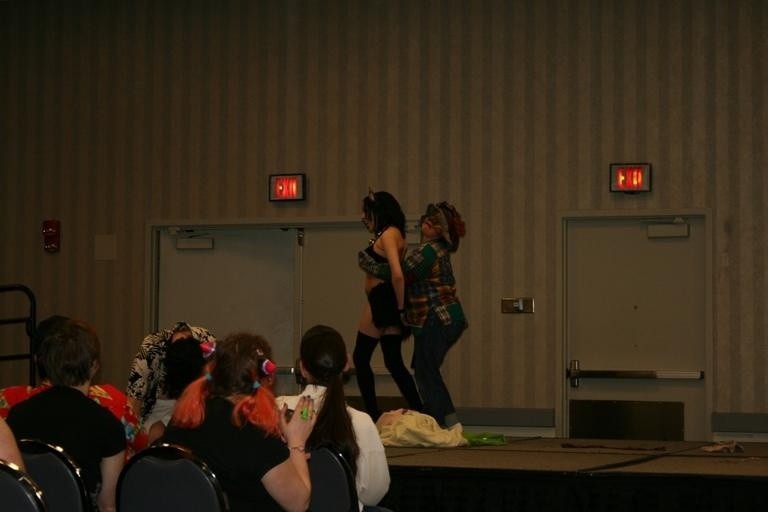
[300,407,310,420]
[308,415,313,419]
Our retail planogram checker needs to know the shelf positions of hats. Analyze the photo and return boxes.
[426,200,466,253]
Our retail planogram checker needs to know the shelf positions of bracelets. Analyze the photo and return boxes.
[288,443,307,452]
[398,308,405,314]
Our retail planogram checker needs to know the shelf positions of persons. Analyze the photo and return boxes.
[273,322,393,512]
[160,331,319,511]
[4,317,126,511]
[353,190,423,424]
[357,199,469,428]
[0,312,148,467]
[127,324,217,446]
